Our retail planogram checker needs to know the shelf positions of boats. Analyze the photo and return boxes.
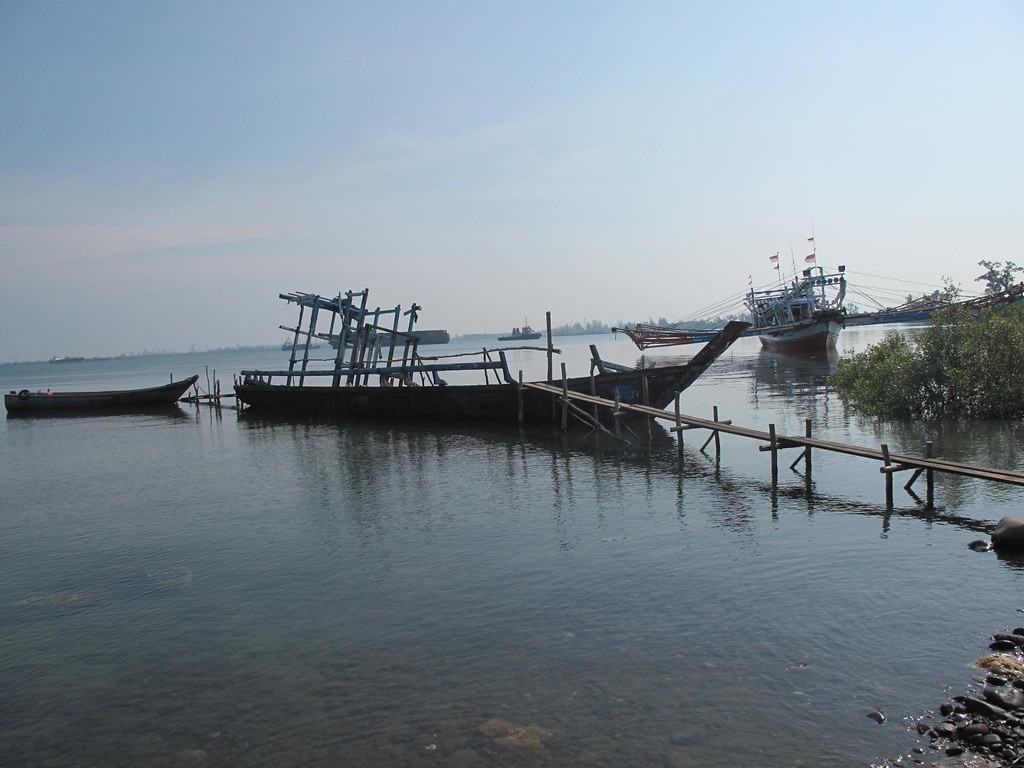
[4,374,199,412]
[497,316,542,341]
[742,216,848,353]
[233,289,753,425]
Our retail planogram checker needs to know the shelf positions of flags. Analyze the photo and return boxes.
[805,255,816,263]
[747,275,751,285]
[770,255,779,270]
[807,237,815,242]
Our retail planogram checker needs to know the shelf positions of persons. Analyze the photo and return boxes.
[45,388,53,395]
